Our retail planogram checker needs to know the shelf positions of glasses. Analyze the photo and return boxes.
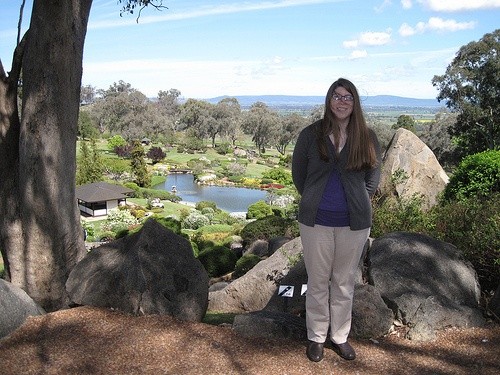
[331,93,355,103]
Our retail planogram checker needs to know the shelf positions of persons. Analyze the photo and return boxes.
[84,230,87,241]
[172,165,177,171]
[291,78,381,362]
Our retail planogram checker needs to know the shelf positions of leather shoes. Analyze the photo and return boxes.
[332,340,358,360]
[307,339,324,363]
